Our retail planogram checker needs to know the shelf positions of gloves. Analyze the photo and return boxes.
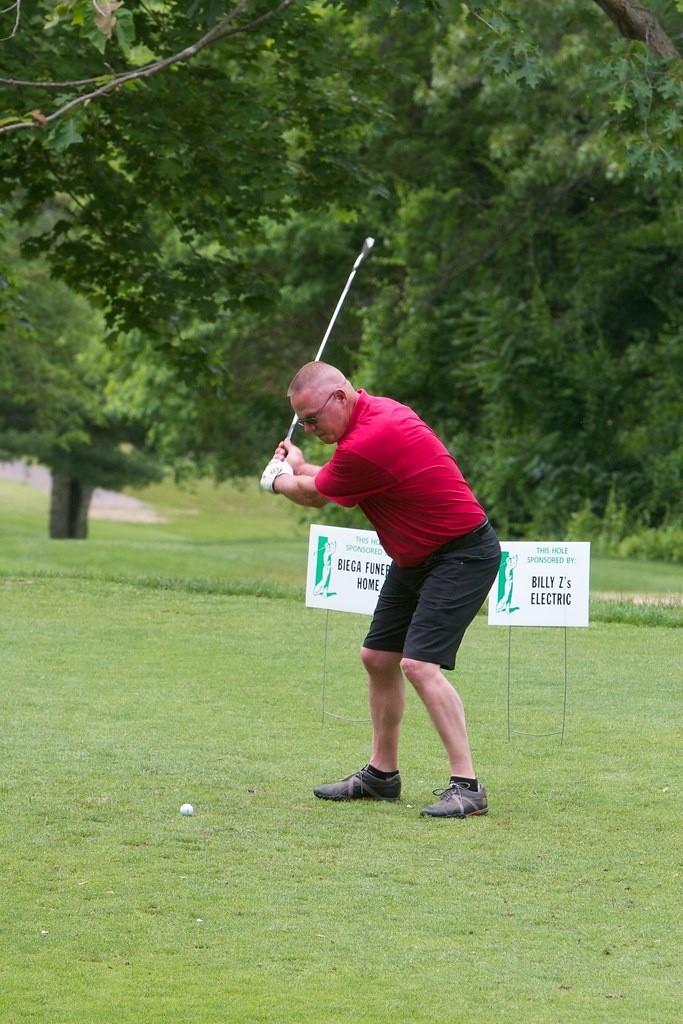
[260,459,293,494]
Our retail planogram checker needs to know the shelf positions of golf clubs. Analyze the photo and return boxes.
[275,235,376,462]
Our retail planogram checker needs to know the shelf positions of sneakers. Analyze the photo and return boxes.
[312,770,402,801]
[420,782,489,818]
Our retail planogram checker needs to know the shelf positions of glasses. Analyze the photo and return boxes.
[298,392,334,426]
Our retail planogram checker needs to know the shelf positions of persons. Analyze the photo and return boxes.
[498,555,518,613]
[259,361,501,819]
[314,541,336,598]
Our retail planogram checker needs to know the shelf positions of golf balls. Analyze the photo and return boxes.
[180,804,193,816]
